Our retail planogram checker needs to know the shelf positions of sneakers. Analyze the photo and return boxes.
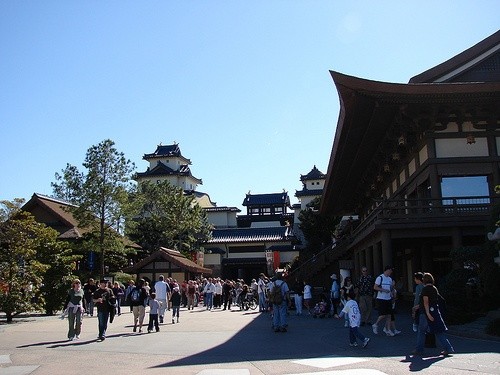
[372,323,379,334]
[383,327,386,333]
[392,329,401,335]
[385,329,395,336]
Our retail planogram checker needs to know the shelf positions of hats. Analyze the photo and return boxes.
[100,278,109,282]
[330,274,337,279]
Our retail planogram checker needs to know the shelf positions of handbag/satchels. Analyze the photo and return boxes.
[373,298,379,309]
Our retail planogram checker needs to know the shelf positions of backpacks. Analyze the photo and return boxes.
[270,280,285,305]
[128,288,143,306]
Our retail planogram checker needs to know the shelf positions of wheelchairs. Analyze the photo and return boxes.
[239,293,258,311]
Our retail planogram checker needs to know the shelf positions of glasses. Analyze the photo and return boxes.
[75,282,80,284]
[347,280,351,282]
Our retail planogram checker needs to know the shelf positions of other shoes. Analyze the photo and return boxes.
[344,321,349,327]
[279,328,288,332]
[361,338,369,348]
[99,336,105,340]
[360,322,365,325]
[349,342,359,347]
[367,321,371,325]
[69,337,73,341]
[441,351,450,355]
[133,315,180,333]
[412,351,421,355]
[272,327,278,332]
[76,334,80,339]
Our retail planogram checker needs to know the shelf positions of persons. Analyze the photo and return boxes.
[126,278,150,333]
[154,275,171,323]
[289,264,402,337]
[80,273,276,316]
[147,292,163,333]
[269,271,291,333]
[334,291,371,349]
[61,279,85,341]
[411,271,455,359]
[92,276,118,341]
[170,287,181,324]
